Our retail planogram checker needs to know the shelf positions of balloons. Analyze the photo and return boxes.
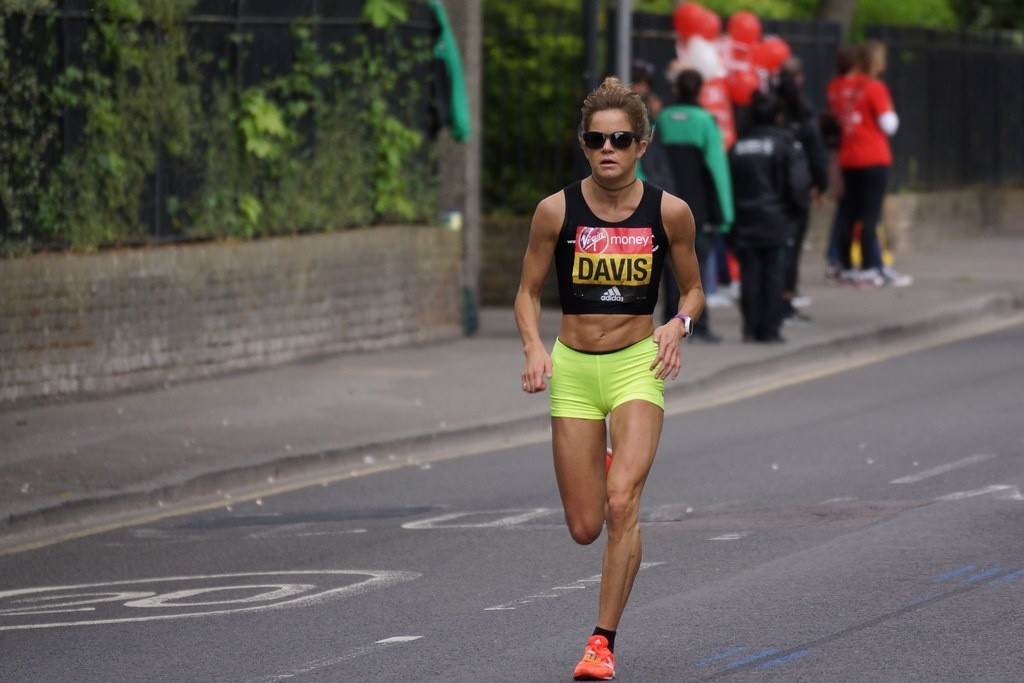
[673,1,789,150]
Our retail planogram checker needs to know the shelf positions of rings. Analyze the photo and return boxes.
[524,383,526,386]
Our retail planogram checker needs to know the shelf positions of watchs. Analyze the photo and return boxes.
[674,314,693,337]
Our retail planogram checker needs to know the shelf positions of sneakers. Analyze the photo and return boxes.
[572,635,616,680]
[605,447,613,478]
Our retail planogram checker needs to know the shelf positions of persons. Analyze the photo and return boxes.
[632,4,909,346]
[513,77,706,680]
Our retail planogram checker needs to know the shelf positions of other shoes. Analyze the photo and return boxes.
[702,332,721,341]
[874,265,912,288]
[841,269,875,283]
[791,296,812,307]
[705,294,732,308]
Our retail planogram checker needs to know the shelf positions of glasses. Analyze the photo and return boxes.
[583,131,640,150]
